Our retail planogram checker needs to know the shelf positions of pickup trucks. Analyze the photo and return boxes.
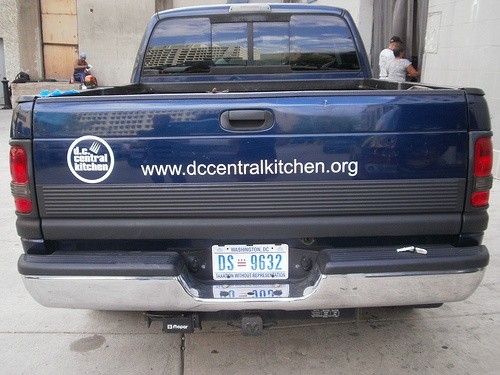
[7,2,493,335]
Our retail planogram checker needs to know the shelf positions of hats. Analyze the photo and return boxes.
[80,51,87,58]
[390,36,404,44]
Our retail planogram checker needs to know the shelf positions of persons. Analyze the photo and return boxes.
[140,115,185,181]
[73,51,94,91]
[385,47,419,84]
[379,36,402,78]
[228,108,286,185]
